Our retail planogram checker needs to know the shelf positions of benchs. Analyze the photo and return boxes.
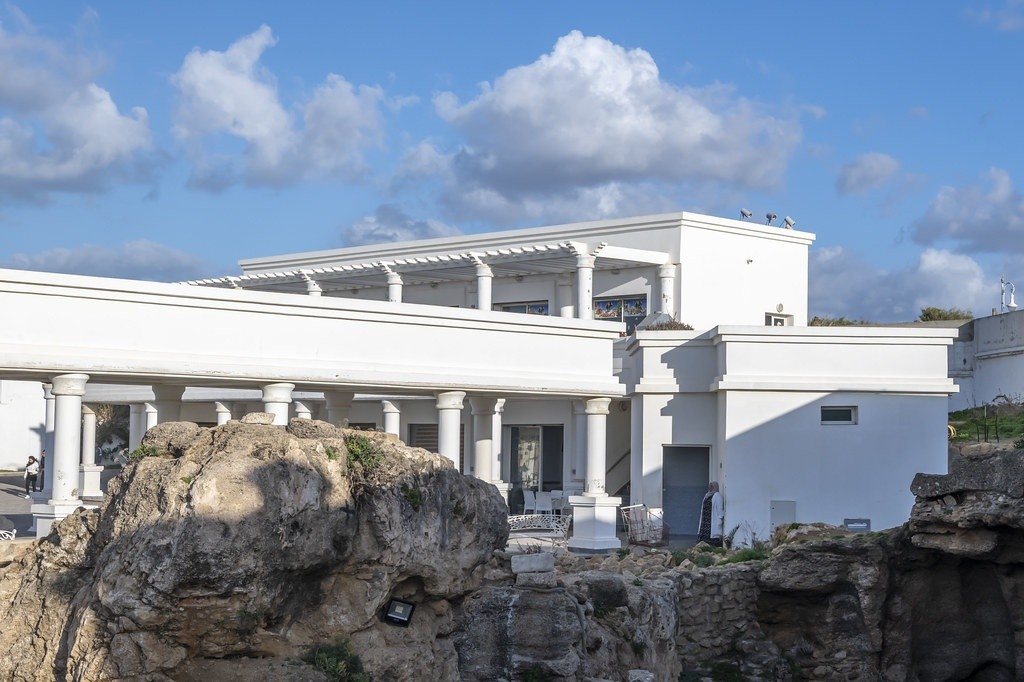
[509,514,572,541]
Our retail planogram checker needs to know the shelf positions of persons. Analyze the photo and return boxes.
[39,450,45,491]
[697,481,724,547]
[24,456,39,500]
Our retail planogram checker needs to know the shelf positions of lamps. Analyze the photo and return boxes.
[740,208,752,221]
[779,216,796,228]
[382,596,417,628]
[1001,278,1018,313]
[767,213,778,225]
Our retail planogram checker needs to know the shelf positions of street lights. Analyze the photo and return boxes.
[1000,277,1018,315]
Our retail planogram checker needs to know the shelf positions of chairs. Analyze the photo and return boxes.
[522,490,575,515]
[976,403,1000,442]
